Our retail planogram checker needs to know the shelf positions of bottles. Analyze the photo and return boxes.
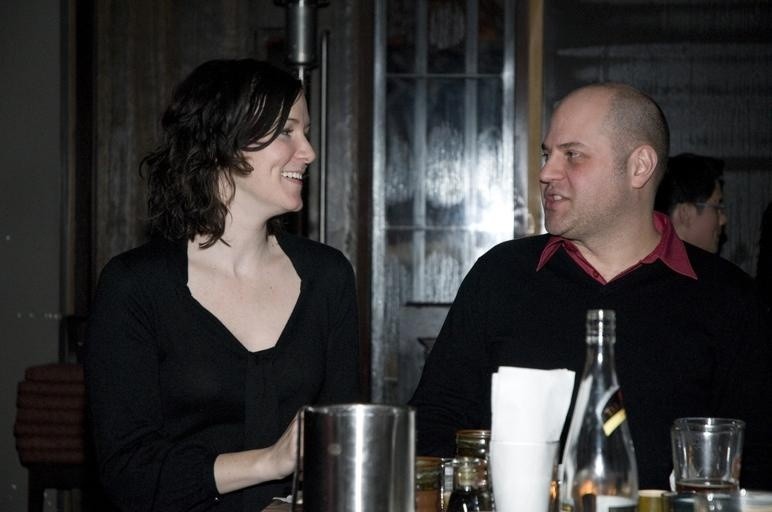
[433,429,493,512]
[559,307,643,511]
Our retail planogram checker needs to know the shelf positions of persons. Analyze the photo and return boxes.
[654,153,728,256]
[85,55,358,512]
[404,84,769,494]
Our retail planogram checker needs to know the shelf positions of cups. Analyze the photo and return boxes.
[489,440,559,511]
[637,417,749,511]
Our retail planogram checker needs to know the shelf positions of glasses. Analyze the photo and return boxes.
[695,201,729,216]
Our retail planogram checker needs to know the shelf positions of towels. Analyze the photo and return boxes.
[484,360,577,512]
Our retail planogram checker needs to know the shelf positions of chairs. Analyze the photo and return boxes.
[416,332,438,362]
[13,355,91,512]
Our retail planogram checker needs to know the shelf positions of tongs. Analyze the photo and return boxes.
[290,403,316,512]
